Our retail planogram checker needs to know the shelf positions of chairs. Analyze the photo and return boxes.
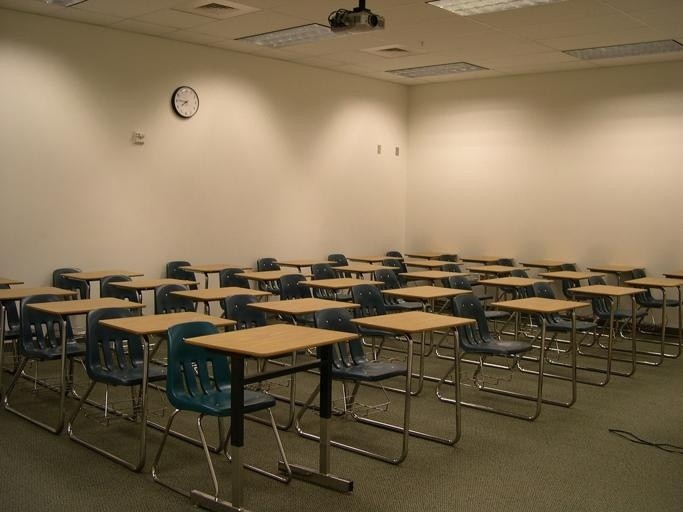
[2,249,683,512]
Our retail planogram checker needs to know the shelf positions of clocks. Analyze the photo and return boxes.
[171,86,199,118]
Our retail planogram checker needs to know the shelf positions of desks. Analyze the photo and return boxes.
[0,275,27,284]
[405,251,458,258]
[169,286,273,301]
[402,259,462,268]
[299,277,385,291]
[99,311,238,336]
[1,284,75,301]
[519,259,577,268]
[459,256,515,263]
[180,264,254,273]
[380,285,471,301]
[399,270,472,280]
[567,284,646,297]
[539,270,606,280]
[587,264,646,272]
[662,271,681,279]
[466,265,531,277]
[108,278,201,291]
[347,255,401,264]
[494,296,591,422]
[478,276,555,287]
[61,267,146,281]
[249,298,361,314]
[27,296,147,318]
[331,264,403,272]
[184,323,354,511]
[234,270,315,280]
[352,309,476,465]
[624,277,682,288]
[273,258,338,268]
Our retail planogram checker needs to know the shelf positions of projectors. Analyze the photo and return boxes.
[331,10,385,33]
